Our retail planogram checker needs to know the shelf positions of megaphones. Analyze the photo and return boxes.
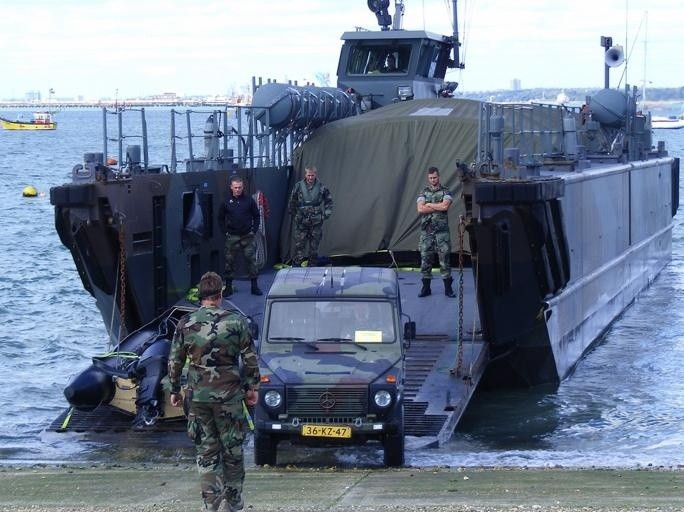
[604,47,625,68]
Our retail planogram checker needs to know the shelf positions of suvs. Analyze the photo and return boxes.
[237,265,417,469]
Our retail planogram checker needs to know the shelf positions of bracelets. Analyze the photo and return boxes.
[248,388,259,392]
[170,391,178,394]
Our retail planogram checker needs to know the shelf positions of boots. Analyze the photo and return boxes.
[442,276,456,298]
[418,278,431,298]
[251,278,263,295]
[222,279,233,297]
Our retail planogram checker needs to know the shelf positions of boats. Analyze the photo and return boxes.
[1,118,59,131]
[44,0,681,466]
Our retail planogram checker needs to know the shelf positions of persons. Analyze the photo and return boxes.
[381,54,402,73]
[416,166,455,297]
[288,164,335,256]
[218,176,263,297]
[167,271,261,512]
